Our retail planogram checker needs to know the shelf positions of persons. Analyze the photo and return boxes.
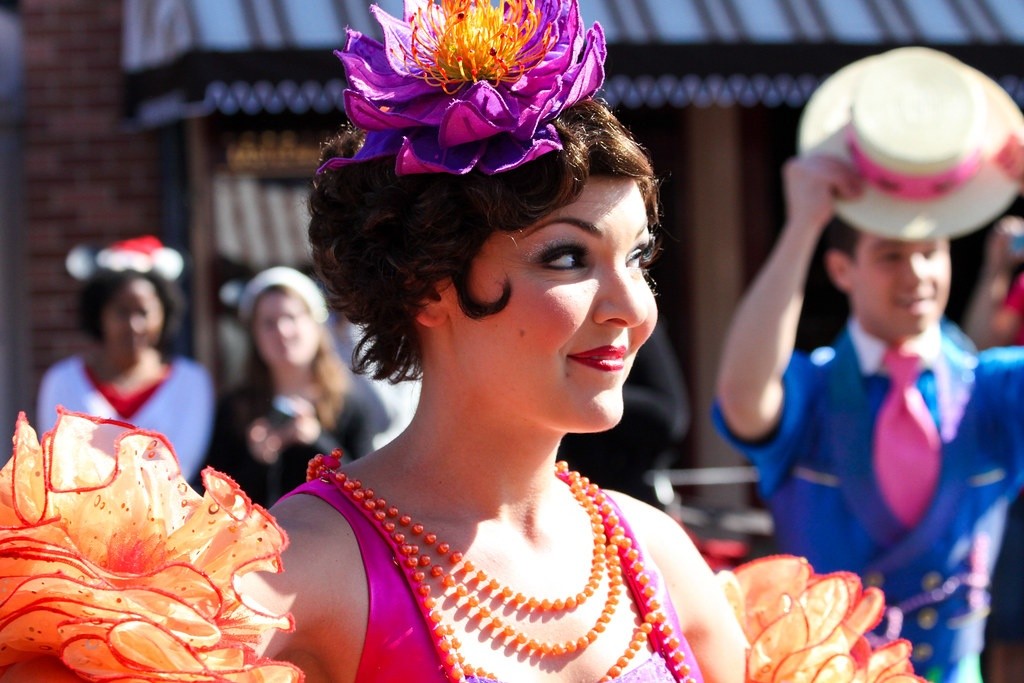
[709,149,1024,683]
[230,98,752,683]
[211,266,375,507]
[33,235,213,491]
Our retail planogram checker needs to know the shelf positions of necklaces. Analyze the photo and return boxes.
[308,446,701,683]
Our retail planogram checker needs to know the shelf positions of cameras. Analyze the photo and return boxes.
[265,398,297,430]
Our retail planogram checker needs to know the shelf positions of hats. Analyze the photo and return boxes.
[96,234,182,282]
[798,46,1024,244]
[236,267,328,325]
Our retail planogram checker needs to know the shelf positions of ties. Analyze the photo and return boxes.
[873,347,941,529]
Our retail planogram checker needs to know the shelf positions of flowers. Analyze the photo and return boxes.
[317,0,608,179]
[0,399,296,683]
[719,553,927,683]
